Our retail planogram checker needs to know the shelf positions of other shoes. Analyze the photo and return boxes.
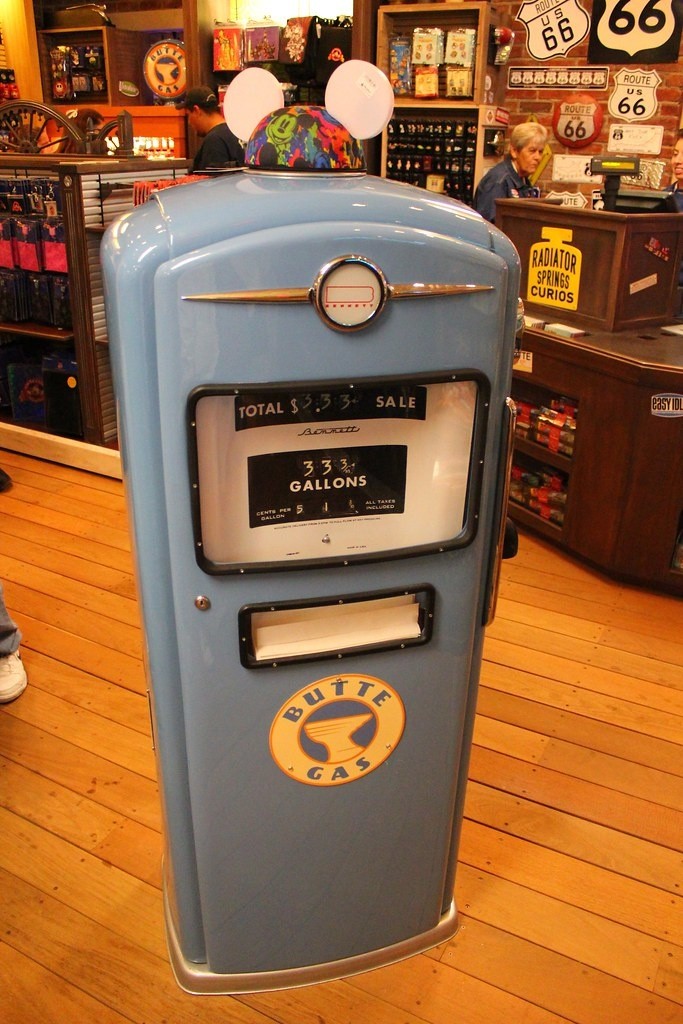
[0,467,12,493]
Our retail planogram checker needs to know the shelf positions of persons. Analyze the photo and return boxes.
[472,122,547,223]
[660,127,683,213]
[0,598,28,703]
[173,85,247,174]
[52,108,105,155]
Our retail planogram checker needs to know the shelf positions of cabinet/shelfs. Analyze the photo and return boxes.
[507,326,682,595]
[374,1,509,205]
[0,1,210,446]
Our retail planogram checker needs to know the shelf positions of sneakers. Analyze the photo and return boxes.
[0,649,27,703]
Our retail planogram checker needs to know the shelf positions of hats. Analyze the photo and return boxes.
[175,86,217,110]
